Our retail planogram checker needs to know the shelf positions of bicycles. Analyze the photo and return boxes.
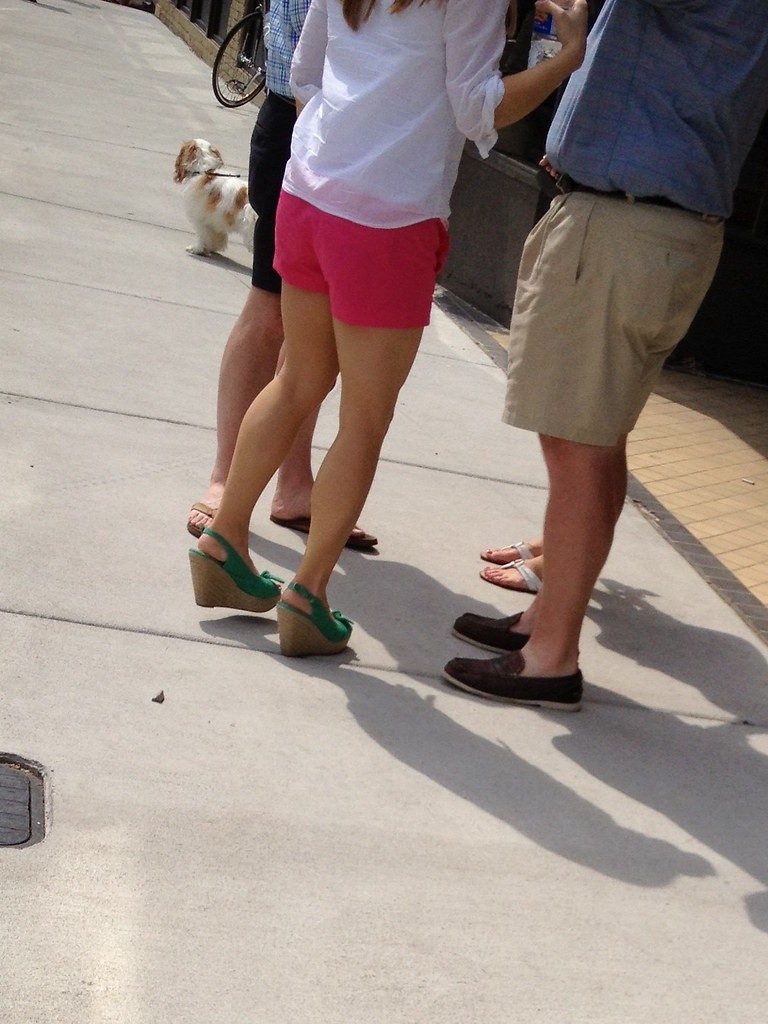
[212,0,269,107]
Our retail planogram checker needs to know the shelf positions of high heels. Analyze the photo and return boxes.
[188,528,285,613]
[277,582,354,656]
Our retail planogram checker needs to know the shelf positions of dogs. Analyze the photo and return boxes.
[169,139,258,255]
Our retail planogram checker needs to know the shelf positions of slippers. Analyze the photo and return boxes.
[481,542,534,565]
[187,502,219,537]
[270,515,378,548]
[480,558,542,594]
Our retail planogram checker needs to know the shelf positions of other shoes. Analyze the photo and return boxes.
[453,612,531,654]
[443,649,583,712]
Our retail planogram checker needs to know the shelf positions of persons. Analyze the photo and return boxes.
[440,0,768,711]
[189,0,588,658]
[188,0,379,550]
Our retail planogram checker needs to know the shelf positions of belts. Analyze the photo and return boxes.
[566,182,694,212]
